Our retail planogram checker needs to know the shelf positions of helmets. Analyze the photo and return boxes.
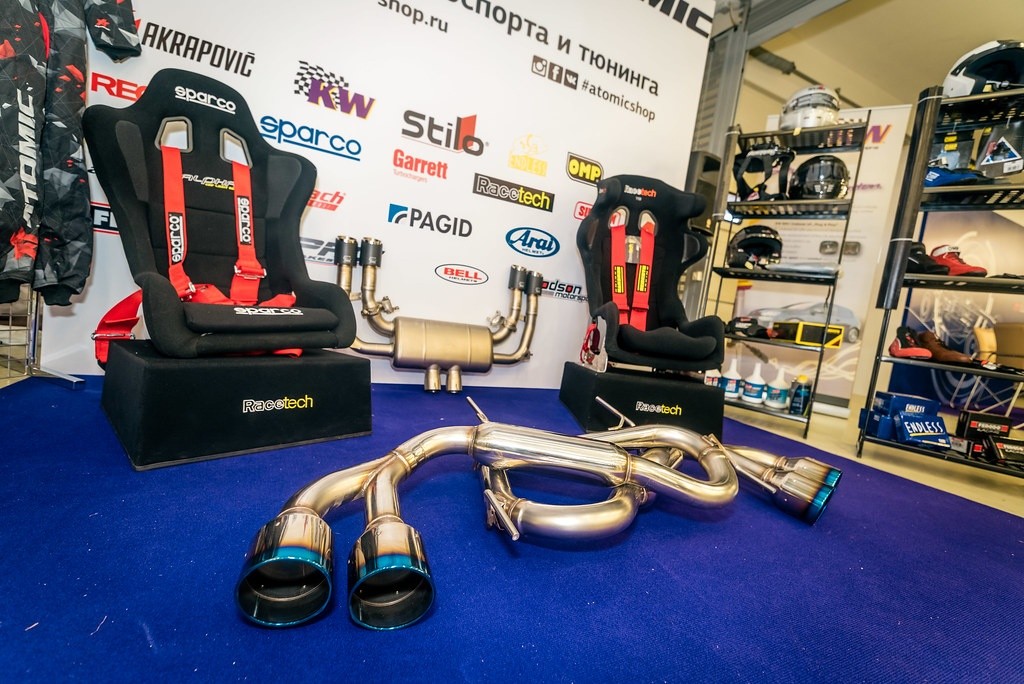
[942,39,1024,99]
[726,225,782,271]
[778,85,840,132]
[789,155,850,199]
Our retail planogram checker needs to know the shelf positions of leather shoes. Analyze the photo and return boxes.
[917,331,971,363]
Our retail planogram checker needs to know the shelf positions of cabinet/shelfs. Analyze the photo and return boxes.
[702,108,875,440]
[856,86,1024,480]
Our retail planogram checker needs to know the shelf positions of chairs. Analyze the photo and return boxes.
[578,174,725,371]
[81,68,357,360]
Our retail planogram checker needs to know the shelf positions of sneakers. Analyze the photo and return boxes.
[889,325,933,360]
[931,244,987,277]
[924,167,995,187]
[904,241,950,274]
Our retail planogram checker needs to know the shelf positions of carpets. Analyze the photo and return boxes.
[1,374,1024,684]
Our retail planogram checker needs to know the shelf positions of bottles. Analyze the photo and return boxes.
[719,357,789,410]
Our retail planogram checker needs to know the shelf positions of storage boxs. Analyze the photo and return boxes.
[771,322,845,350]
[858,391,1024,467]
